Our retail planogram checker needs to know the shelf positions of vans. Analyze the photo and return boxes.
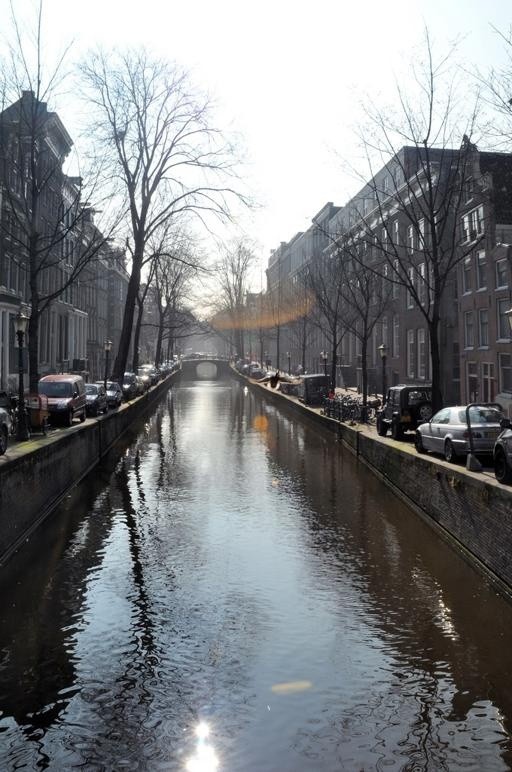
[37,374,87,427]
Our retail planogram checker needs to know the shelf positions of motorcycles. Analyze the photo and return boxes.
[323,392,381,424]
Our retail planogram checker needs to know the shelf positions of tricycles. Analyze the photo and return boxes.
[2,391,50,441]
[299,374,334,405]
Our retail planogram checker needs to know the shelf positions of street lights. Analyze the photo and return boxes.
[377,343,390,405]
[287,351,291,375]
[105,340,113,390]
[11,310,30,441]
[320,348,330,396]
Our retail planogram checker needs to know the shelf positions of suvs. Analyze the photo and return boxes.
[376,384,433,441]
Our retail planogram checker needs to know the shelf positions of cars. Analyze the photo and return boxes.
[84,354,178,417]
[493,419,512,484]
[415,405,512,463]
[235,358,265,377]
[0,407,12,456]
